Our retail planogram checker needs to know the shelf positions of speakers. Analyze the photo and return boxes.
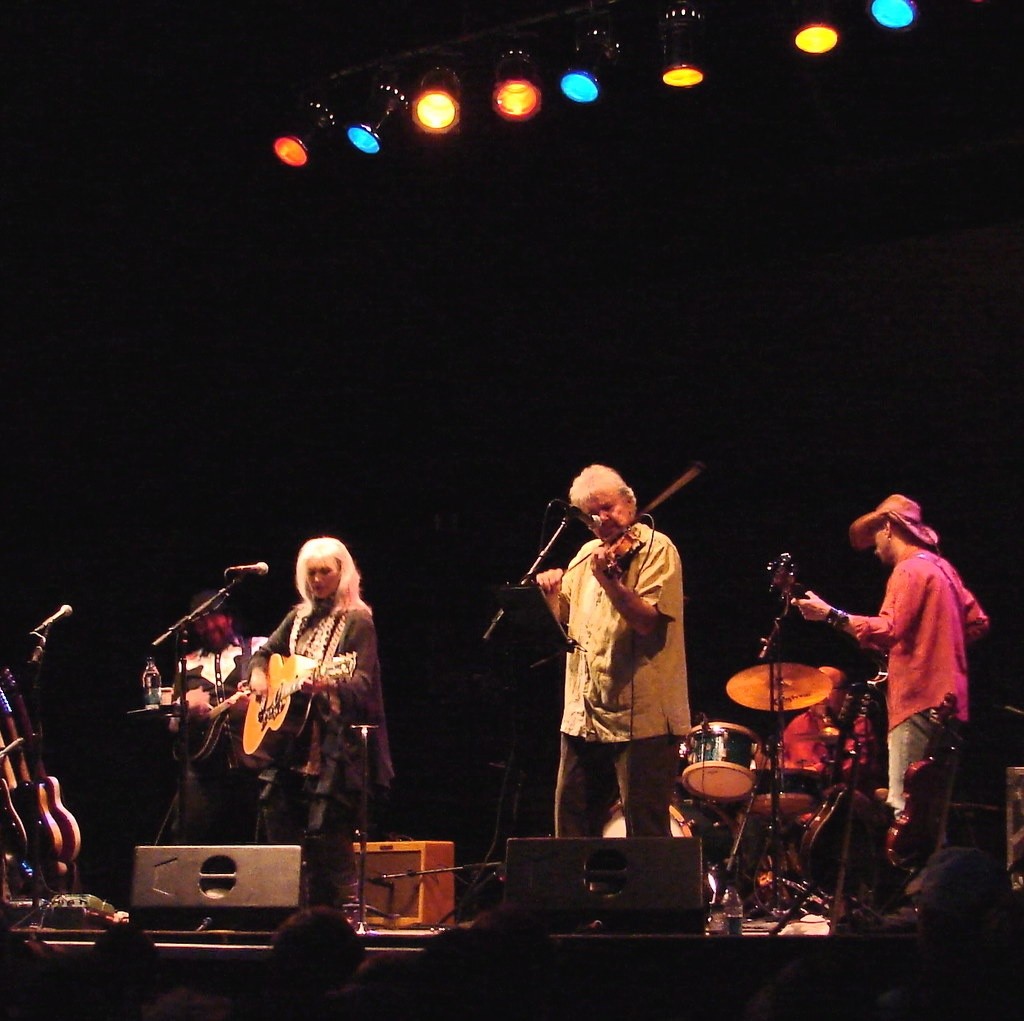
[352,839,455,929]
[505,836,704,937]
[131,843,310,945]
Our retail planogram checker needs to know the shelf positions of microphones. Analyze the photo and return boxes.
[34,604,73,632]
[557,498,603,531]
[228,561,270,575]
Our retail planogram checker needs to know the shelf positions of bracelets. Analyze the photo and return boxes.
[825,606,849,632]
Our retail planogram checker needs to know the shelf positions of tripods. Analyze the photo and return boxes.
[737,596,831,923]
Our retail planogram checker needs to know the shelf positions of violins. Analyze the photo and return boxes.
[885,691,959,871]
[605,528,646,580]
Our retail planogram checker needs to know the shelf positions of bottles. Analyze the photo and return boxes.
[142,657,162,709]
[721,880,743,934]
[345,895,361,930]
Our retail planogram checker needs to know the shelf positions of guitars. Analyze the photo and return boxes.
[798,682,873,898]
[242,651,358,761]
[172,679,252,762]
[767,552,889,697]
[0,667,81,876]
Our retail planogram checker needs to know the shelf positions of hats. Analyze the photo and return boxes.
[849,495,939,551]
[187,590,234,622]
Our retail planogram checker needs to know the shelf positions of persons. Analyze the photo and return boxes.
[751,847,1024,1021]
[0,908,565,1020]
[245,539,394,915]
[788,495,989,917]
[170,600,270,844]
[778,666,894,910]
[536,464,691,838]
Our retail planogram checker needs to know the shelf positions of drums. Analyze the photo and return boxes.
[745,768,820,815]
[601,796,730,872]
[679,722,758,801]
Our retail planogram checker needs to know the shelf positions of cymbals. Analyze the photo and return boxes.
[726,662,833,711]
